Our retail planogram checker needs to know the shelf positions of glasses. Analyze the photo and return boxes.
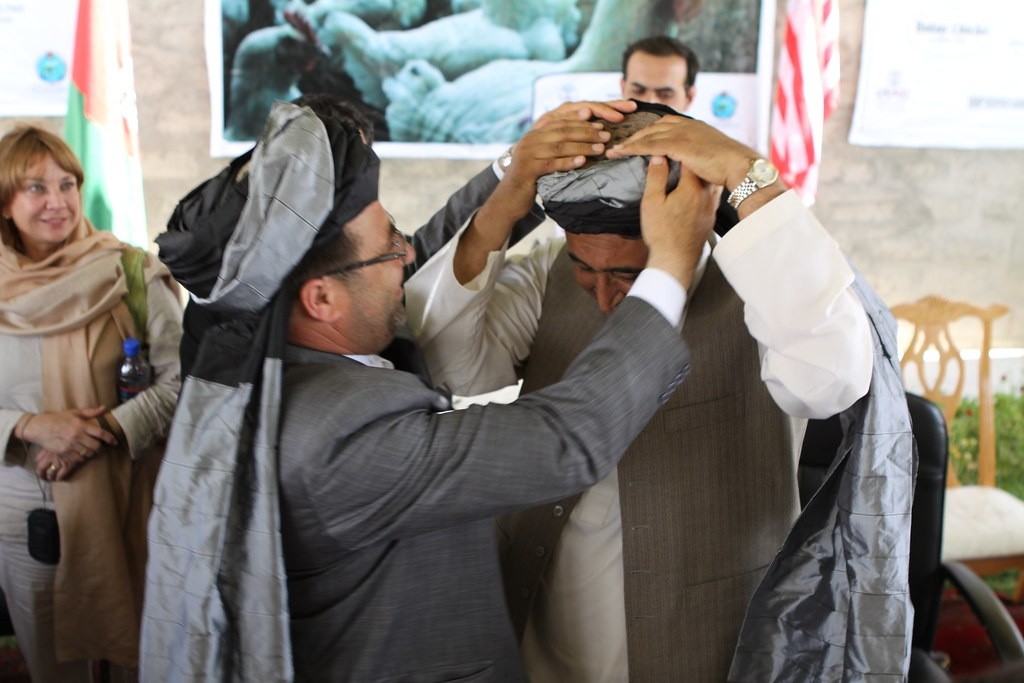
[319,212,406,284]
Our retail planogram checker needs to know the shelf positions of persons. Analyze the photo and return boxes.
[0,127,185,683]
[404,99,873,683]
[138,98,724,683]
[621,34,701,114]
[278,10,393,139]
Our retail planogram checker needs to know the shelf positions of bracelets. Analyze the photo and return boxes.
[95,414,116,441]
[499,146,514,170]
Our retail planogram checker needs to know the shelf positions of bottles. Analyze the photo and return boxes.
[119,339,151,404]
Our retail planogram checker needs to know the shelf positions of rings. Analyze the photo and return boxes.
[48,464,57,472]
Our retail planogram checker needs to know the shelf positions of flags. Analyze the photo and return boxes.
[767,0,841,212]
[65,0,151,251]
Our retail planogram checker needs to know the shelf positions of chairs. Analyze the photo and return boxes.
[906,389,1024,683]
[888,296,1024,597]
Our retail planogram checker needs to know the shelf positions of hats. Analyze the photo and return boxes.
[536,97,915,683]
[138,97,381,683]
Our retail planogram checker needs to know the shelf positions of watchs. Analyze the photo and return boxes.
[725,158,781,210]
